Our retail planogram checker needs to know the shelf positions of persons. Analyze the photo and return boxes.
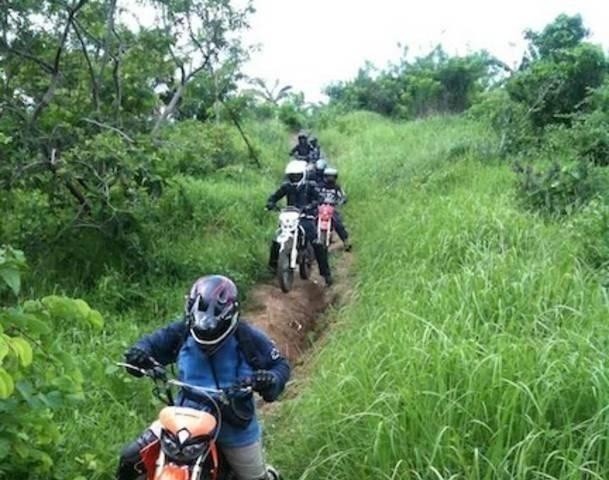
[287,132,327,183]
[311,167,352,251]
[117,273,291,480]
[264,159,334,285]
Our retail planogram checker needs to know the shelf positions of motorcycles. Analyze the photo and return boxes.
[116,351,278,479]
[266,151,340,294]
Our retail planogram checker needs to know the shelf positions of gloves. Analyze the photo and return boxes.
[125,347,153,378]
[251,369,276,392]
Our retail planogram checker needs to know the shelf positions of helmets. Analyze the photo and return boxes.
[284,133,339,188]
[185,274,239,345]
